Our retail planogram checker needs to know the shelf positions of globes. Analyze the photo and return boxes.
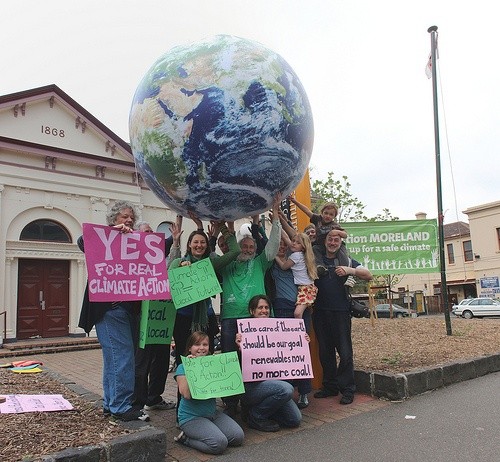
[128,34,314,222]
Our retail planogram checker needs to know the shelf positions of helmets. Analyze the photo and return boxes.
[350,298,369,318]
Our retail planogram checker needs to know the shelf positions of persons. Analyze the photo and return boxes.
[219,294,311,432]
[451,296,458,315]
[166,191,374,406]
[77,200,149,420]
[169,219,241,428]
[134,214,183,410]
[173,331,244,455]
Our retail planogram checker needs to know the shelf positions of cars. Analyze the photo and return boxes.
[368,303,419,319]
[452,297,500,320]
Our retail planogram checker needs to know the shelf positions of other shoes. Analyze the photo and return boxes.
[223,399,239,417]
[173,430,187,443]
[315,388,339,398]
[339,391,354,404]
[103,407,144,422]
[297,394,308,407]
[143,400,175,410]
[246,410,281,432]
